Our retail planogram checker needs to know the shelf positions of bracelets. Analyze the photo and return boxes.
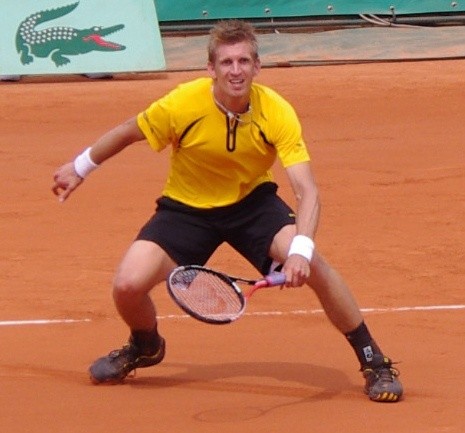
[74,146,99,180]
[287,235,315,263]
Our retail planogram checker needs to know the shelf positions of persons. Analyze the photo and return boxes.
[51,20,403,403]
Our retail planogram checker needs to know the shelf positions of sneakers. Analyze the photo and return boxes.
[363,353,403,402]
[88,335,166,383]
